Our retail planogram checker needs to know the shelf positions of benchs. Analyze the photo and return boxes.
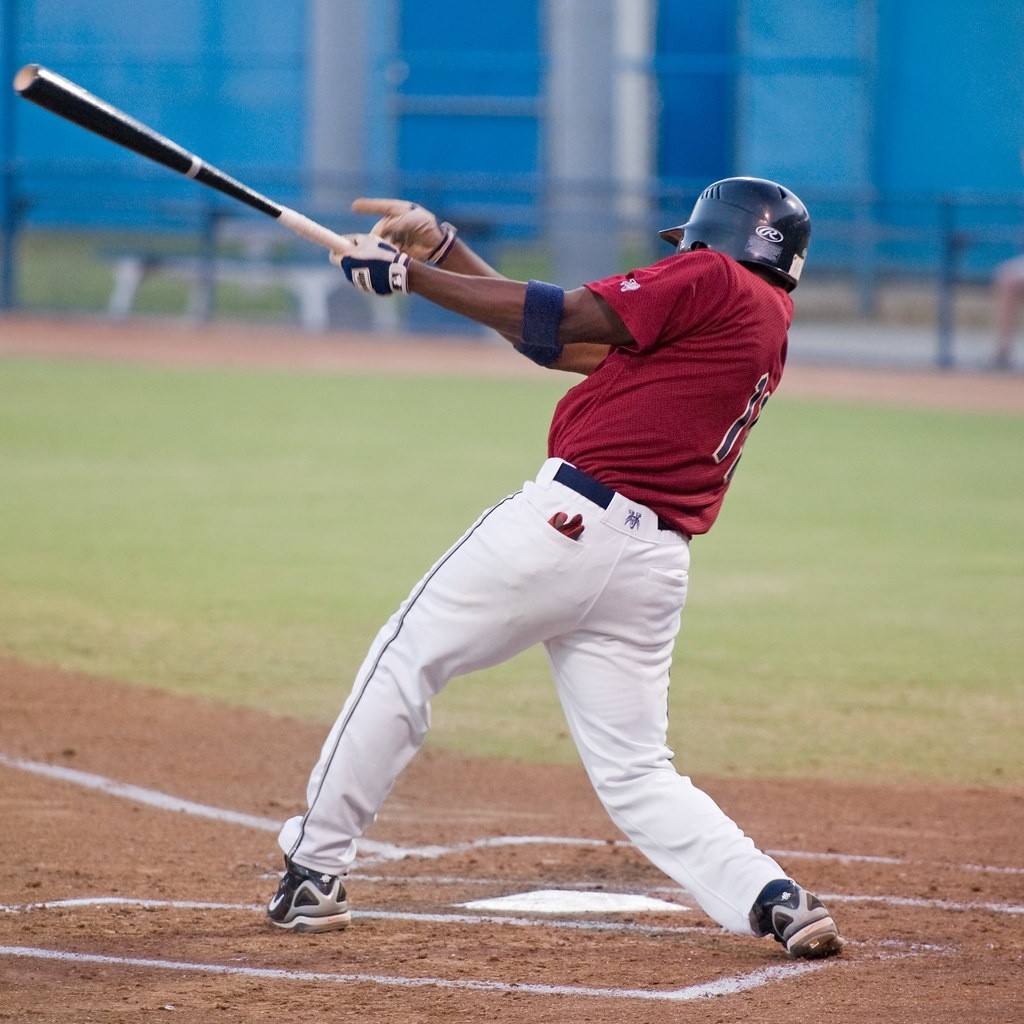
[98,208,499,335]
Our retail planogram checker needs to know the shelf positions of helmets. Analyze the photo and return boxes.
[658,174,812,293]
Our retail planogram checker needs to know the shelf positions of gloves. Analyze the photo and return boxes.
[328,233,407,295]
[352,197,457,271]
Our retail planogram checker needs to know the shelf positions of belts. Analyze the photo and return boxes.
[552,461,675,536]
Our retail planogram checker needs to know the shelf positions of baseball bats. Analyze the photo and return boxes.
[13,61,359,256]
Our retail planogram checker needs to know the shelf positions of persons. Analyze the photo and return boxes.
[975,254,1024,371]
[266,179,841,958]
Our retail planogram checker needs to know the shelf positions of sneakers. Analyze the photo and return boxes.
[268,855,352,933]
[754,880,847,961]
